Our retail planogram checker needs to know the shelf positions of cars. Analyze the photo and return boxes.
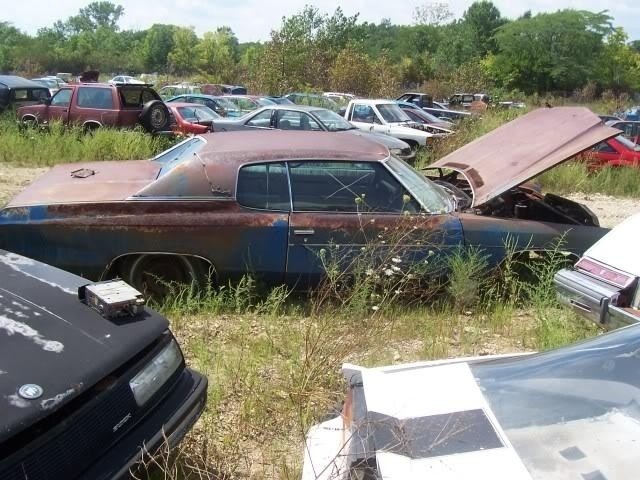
[155,81,515,168]
[550,213,640,333]
[0,249,208,480]
[107,75,146,86]
[0,70,100,121]
[0,104,624,308]
[570,104,640,176]
[307,322,640,480]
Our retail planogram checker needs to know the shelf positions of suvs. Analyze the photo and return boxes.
[15,83,176,145]
